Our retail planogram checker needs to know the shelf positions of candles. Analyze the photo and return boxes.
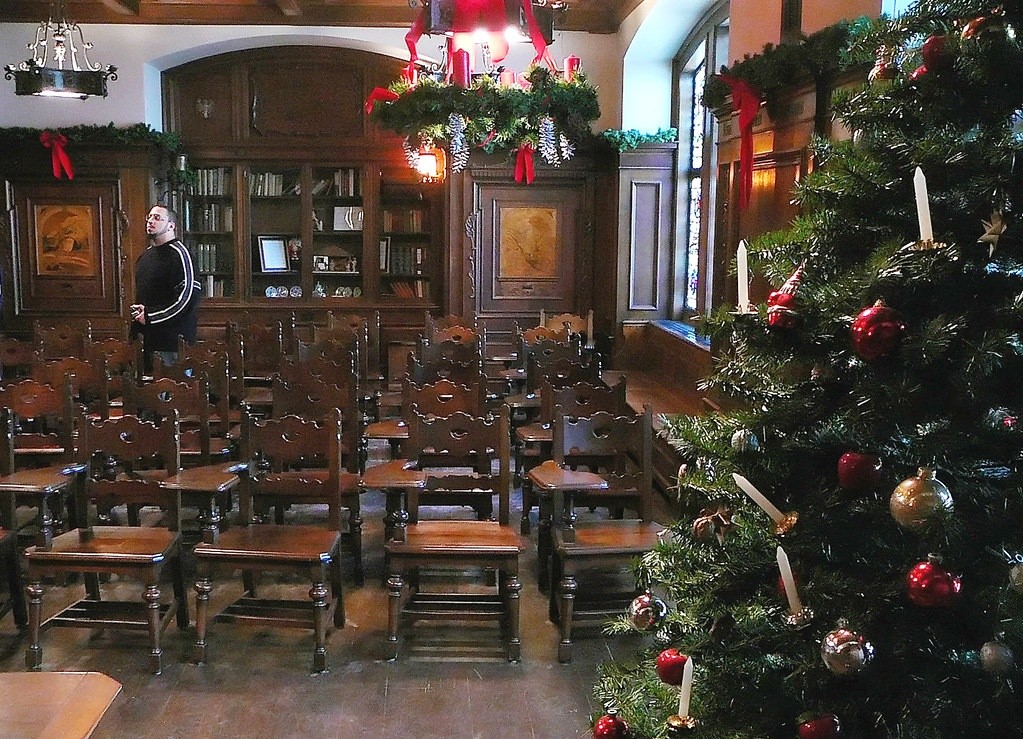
[732,472,786,525]
[776,546,802,616]
[912,166,935,242]
[737,240,750,313]
[678,656,693,719]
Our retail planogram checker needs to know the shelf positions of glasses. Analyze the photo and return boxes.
[145,214,169,222]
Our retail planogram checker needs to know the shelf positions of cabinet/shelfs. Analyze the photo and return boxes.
[181,154,432,416]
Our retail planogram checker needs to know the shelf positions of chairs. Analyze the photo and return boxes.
[2,306,678,668]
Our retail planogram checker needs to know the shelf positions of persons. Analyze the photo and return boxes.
[128,205,201,401]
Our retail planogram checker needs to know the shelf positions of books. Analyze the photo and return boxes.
[380,209,429,298]
[249,169,363,196]
[185,168,233,298]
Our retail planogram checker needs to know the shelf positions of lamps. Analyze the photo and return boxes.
[3,0,118,102]
